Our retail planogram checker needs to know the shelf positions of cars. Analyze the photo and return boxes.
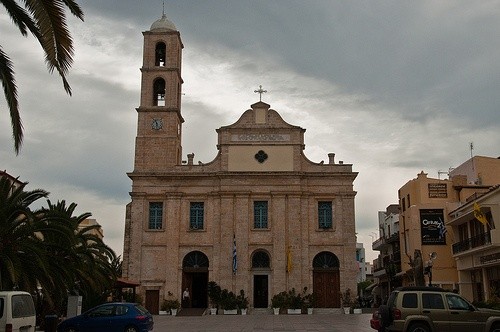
[370,310,383,330]
[56,301,155,332]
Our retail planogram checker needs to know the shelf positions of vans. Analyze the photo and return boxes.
[0,290,36,332]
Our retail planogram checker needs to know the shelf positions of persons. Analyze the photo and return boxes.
[107,295,117,301]
[183,288,190,308]
[410,249,425,285]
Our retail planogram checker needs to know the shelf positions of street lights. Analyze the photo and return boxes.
[429,252,438,286]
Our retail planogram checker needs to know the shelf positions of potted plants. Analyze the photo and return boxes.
[207,280,251,315]
[158,300,181,316]
[270,286,318,315]
[341,291,364,314]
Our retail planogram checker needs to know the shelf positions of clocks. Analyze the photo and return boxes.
[151,118,163,130]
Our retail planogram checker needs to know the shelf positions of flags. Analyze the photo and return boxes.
[233,239,237,272]
[288,253,291,272]
[474,203,486,225]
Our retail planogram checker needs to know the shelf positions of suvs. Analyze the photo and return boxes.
[377,290,500,332]
